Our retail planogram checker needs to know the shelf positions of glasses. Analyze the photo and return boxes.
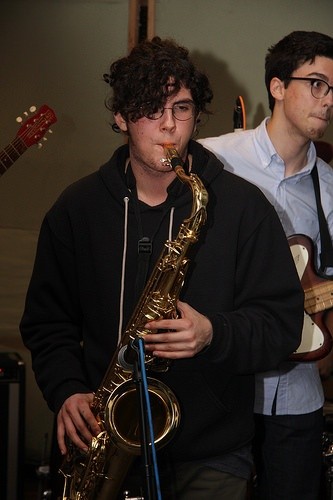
[286,76,333,97]
[121,101,197,121]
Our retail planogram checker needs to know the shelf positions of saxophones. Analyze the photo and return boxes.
[53,141,209,500]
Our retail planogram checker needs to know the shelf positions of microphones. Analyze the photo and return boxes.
[117,337,145,369]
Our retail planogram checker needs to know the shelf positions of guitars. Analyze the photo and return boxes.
[282,234,333,363]
[0,104,58,179]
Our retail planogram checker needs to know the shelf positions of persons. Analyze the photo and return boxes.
[190,29,333,500]
[17,33,307,500]
